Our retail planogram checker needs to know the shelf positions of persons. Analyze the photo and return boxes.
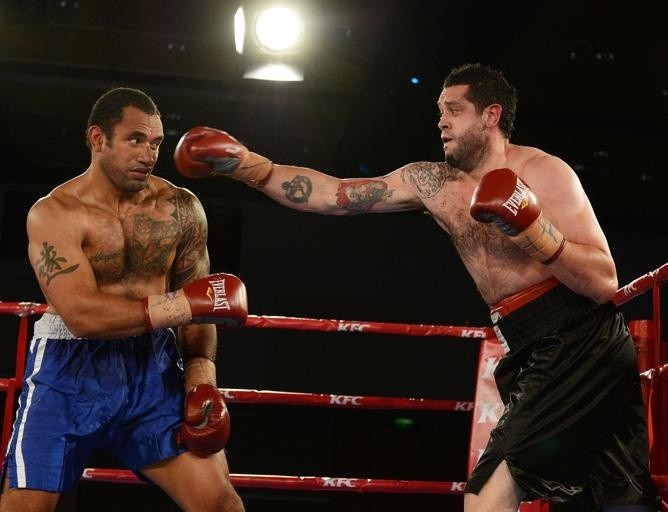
[1,87,246,511]
[172,63,662,511]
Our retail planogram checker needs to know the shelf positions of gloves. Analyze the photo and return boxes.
[467,169,566,265]
[174,127,274,190]
[179,357,232,457]
[142,272,249,333]
